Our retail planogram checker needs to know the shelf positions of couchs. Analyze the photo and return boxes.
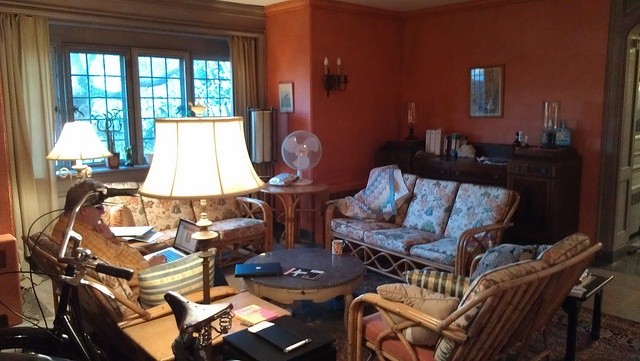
[103,182,273,263]
[325,165,519,283]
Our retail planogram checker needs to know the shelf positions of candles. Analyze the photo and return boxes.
[323,56,328,73]
[547,119,553,128]
[410,109,413,124]
[336,58,342,76]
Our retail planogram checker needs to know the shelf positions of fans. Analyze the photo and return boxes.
[281,129,323,185]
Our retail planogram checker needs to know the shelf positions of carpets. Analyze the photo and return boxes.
[268,273,640,360]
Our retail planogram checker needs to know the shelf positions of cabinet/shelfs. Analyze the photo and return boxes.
[375,141,426,177]
[508,144,582,240]
[412,157,507,186]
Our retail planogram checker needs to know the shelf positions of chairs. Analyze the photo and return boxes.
[21,230,241,331]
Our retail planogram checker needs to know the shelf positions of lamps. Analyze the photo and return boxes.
[46,121,113,180]
[138,116,280,360]
[246,106,277,218]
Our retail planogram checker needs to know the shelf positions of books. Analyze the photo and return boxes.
[230,305,277,327]
[282,267,325,279]
[425,128,469,156]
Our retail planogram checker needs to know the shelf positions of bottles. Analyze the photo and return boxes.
[512,132,522,158]
[519,132,524,146]
[522,136,529,148]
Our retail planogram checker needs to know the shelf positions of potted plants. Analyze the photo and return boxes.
[93,107,129,169]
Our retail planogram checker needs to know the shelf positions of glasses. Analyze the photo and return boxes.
[93,204,103,211]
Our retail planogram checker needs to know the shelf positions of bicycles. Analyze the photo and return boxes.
[1,180,237,360]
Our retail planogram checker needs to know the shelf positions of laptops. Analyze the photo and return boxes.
[142,218,202,267]
[235,261,283,278]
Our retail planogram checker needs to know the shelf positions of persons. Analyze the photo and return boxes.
[52,180,229,298]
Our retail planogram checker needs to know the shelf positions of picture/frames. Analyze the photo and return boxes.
[467,66,505,119]
[278,80,294,114]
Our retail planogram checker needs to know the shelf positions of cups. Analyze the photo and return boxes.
[332,239,345,255]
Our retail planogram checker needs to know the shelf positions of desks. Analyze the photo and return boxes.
[123,289,338,360]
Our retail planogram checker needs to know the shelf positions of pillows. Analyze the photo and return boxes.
[139,247,218,310]
[107,203,136,227]
[380,282,457,350]
[402,269,471,299]
[467,244,553,280]
